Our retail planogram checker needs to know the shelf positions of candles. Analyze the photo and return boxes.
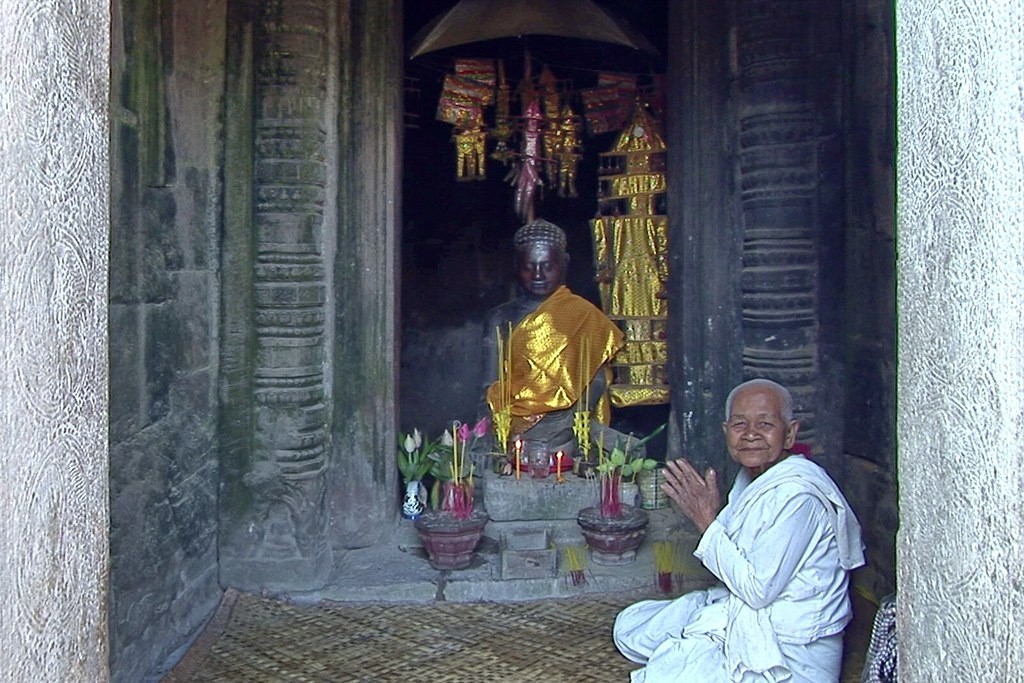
[516,440,520,480]
[556,450,563,482]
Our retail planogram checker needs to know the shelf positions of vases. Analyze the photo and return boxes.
[402,482,425,521]
[431,481,441,511]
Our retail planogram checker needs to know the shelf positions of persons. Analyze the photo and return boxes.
[612,380,865,683]
[482,217,626,457]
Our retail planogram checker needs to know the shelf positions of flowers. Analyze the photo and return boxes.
[396,427,432,478]
[444,420,489,472]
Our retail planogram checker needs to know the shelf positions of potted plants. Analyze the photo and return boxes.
[613,422,672,508]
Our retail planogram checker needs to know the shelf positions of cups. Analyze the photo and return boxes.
[527,443,550,478]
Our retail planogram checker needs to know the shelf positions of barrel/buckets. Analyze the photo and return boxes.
[636,461,670,511]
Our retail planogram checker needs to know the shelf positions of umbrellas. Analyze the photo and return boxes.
[406,0,662,114]
